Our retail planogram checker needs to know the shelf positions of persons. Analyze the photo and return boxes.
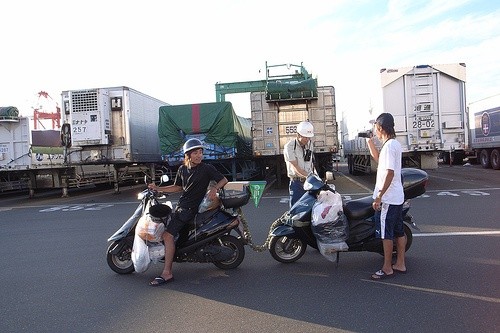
[283,121,317,209]
[366,112,406,279]
[146,138,228,285]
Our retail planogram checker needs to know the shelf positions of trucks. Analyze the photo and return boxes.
[342,62,470,175]
[158,101,251,180]
[250,85,338,181]
[468,95,500,170]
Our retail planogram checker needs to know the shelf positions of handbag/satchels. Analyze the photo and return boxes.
[131,200,165,272]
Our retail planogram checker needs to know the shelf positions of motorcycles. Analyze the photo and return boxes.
[106,174,251,275]
[268,167,430,264]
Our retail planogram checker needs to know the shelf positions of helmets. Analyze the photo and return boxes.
[296,122,315,137]
[149,204,172,223]
[183,138,205,153]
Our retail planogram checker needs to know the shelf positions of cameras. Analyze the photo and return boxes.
[358,130,373,138]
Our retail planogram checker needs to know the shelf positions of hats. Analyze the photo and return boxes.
[375,113,395,126]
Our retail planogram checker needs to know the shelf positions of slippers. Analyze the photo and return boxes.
[391,263,408,274]
[371,269,395,280]
[149,276,175,286]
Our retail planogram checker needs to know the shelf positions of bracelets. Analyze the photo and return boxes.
[306,171,312,178]
[213,186,218,190]
